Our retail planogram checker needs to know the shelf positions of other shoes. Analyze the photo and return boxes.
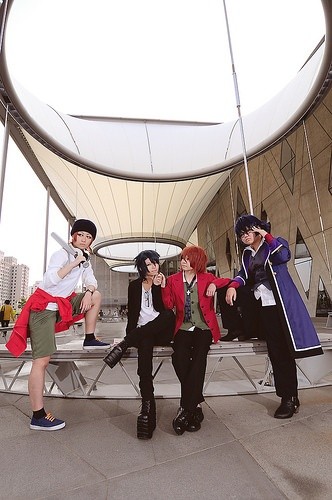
[220,327,242,341]
[274,394,300,419]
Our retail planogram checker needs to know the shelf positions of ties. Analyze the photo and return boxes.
[184,274,196,323]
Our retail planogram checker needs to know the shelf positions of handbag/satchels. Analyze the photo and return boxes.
[0,305,5,322]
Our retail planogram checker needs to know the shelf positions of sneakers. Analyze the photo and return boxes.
[83,338,110,349]
[185,407,204,432]
[172,407,192,435]
[29,413,65,431]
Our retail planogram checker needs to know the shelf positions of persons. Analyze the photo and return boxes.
[102,248,177,439]
[159,244,231,436]
[115,307,119,320]
[0,300,14,337]
[107,309,111,315]
[6,218,112,431]
[99,310,103,320]
[217,213,325,419]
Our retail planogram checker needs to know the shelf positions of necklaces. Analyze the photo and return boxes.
[185,274,194,295]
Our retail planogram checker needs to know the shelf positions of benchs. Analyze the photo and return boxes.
[0,333,332,400]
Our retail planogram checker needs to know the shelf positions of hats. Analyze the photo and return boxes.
[71,219,96,239]
[4,300,10,304]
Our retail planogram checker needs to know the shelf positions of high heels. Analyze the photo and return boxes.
[103,345,126,369]
[136,396,156,439]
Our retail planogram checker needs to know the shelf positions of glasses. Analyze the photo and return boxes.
[240,228,251,238]
[144,292,149,307]
